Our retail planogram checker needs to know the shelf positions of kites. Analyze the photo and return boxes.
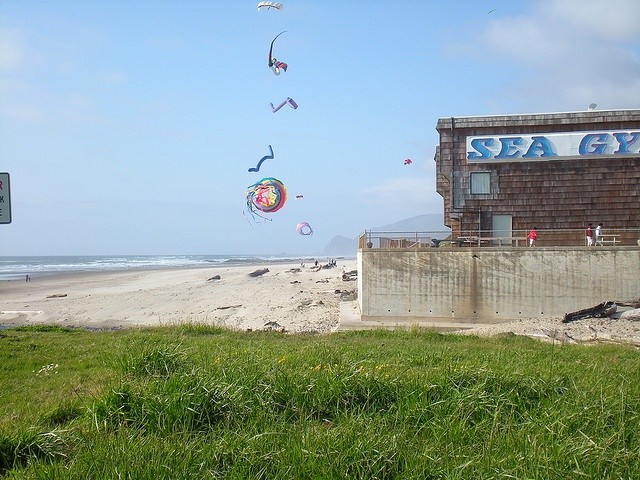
[269,97,298,113]
[296,222,313,237]
[241,177,288,226]
[257,1,283,12]
[248,144,274,172]
[296,195,304,199]
[404,159,412,165]
[268,30,288,76]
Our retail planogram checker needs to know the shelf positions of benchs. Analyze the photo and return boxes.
[450,241,474,248]
[596,240,621,246]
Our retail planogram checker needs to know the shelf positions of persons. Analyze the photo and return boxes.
[586,222,593,246]
[342,266,345,275]
[315,260,318,266]
[527,227,538,247]
[595,223,604,246]
[329,258,336,265]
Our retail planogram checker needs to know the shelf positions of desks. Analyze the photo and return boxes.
[597,234,621,241]
[457,236,479,240]
